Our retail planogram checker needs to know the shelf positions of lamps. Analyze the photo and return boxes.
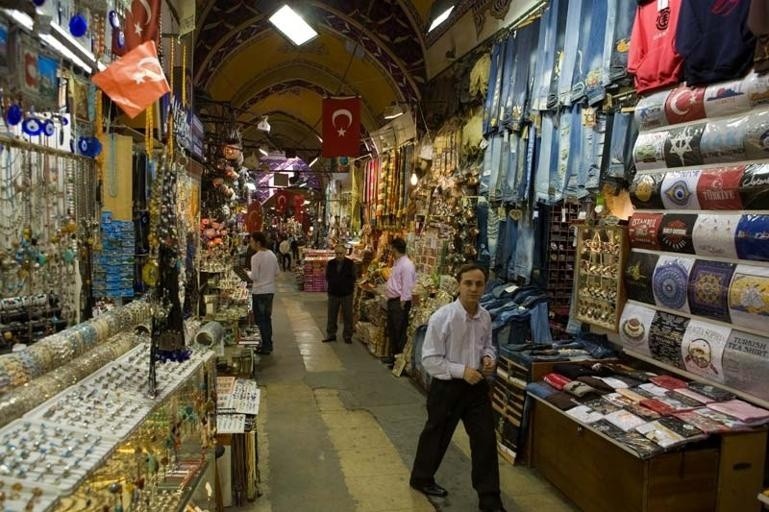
[380,96,448,119]
[411,103,418,186]
[257,114,273,131]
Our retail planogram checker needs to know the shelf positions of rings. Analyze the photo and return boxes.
[217,377,259,434]
[36,385,155,438]
[2,483,56,509]
[87,363,175,397]
[130,460,200,512]
[0,420,118,487]
[127,343,207,377]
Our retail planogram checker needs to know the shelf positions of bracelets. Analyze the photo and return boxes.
[0,293,155,420]
[184,315,224,351]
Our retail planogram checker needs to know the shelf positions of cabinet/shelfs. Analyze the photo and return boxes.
[529,364,718,511]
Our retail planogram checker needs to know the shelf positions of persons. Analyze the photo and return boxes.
[379,238,416,370]
[280,238,293,273]
[266,232,274,252]
[276,239,283,265]
[291,238,300,259]
[287,234,294,247]
[410,264,512,512]
[243,232,280,355]
[243,235,255,270]
[320,245,357,346]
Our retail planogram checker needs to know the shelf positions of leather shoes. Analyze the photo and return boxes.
[382,358,394,363]
[322,337,336,342]
[406,480,450,500]
[388,365,394,370]
[344,336,352,344]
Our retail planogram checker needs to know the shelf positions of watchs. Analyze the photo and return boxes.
[577,224,625,325]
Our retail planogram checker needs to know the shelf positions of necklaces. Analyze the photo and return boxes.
[230,430,263,507]
[53,363,222,512]
[181,44,190,111]
[156,18,165,60]
[104,103,199,395]
[168,34,178,95]
[0,39,109,275]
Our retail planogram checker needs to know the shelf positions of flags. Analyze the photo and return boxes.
[91,39,171,119]
[321,95,363,159]
[112,0,160,54]
[274,190,288,213]
[246,202,264,233]
